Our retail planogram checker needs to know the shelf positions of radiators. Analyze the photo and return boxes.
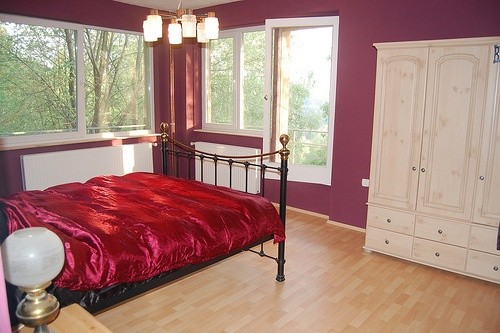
[190,142,261,195]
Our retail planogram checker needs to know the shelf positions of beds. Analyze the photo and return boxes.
[0,123,292,333]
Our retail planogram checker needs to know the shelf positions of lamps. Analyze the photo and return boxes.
[4,227,66,333]
[144,0,220,45]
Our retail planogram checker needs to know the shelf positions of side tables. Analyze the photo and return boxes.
[14,303,114,333]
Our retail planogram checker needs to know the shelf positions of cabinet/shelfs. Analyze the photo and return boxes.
[361,37,500,281]
